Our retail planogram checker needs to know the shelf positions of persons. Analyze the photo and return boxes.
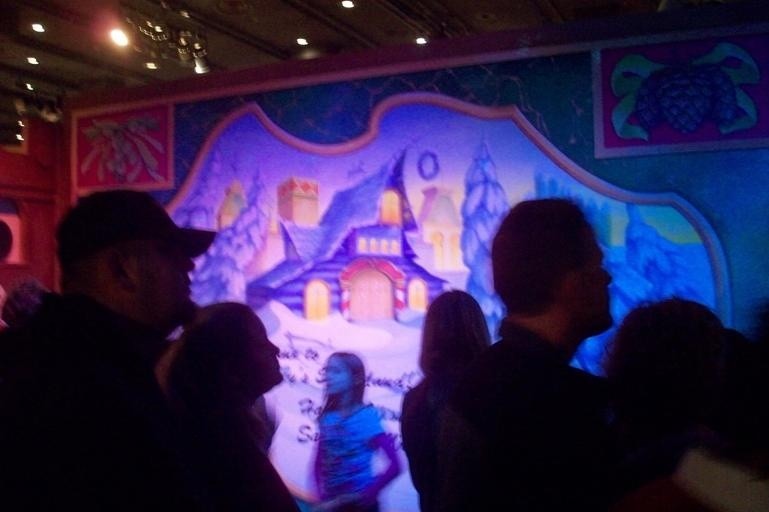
[439,196,768,511]
[595,289,768,511]
[307,351,403,512]
[399,288,499,511]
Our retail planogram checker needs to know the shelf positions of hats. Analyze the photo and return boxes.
[56,189,217,261]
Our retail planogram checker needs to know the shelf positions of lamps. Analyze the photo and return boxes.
[178,11,213,75]
